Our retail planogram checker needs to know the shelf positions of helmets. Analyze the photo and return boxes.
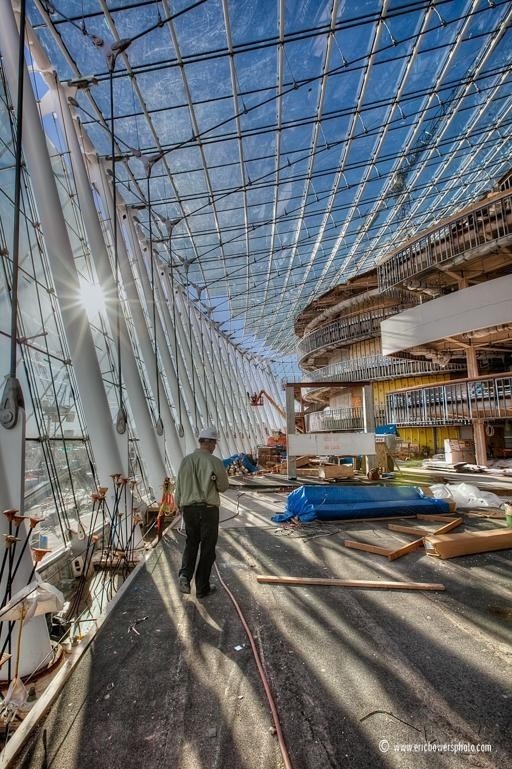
[199,427,219,439]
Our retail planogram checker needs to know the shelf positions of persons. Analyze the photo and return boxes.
[251,391,258,404]
[174,426,229,598]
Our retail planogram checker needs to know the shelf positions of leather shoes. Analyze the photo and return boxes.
[179,572,191,594]
[196,583,216,598]
